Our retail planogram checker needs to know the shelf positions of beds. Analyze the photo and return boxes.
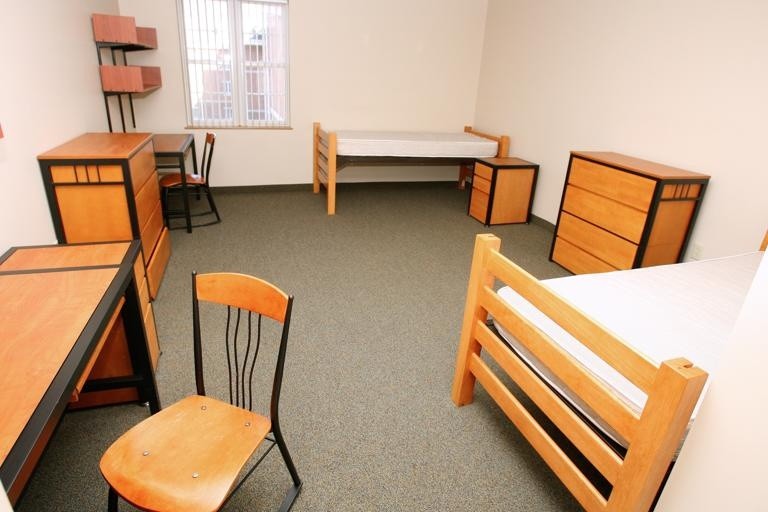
[307,121,510,217]
[446,226,766,512]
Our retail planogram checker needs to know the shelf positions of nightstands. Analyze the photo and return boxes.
[468,155,538,228]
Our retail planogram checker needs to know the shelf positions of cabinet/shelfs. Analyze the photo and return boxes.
[89,10,160,94]
[549,151,713,277]
[38,132,172,301]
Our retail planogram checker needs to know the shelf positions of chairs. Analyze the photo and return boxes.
[101,270,303,509]
[161,132,223,234]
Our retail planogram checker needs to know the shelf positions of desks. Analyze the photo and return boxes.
[0,242,162,511]
[148,133,198,233]
